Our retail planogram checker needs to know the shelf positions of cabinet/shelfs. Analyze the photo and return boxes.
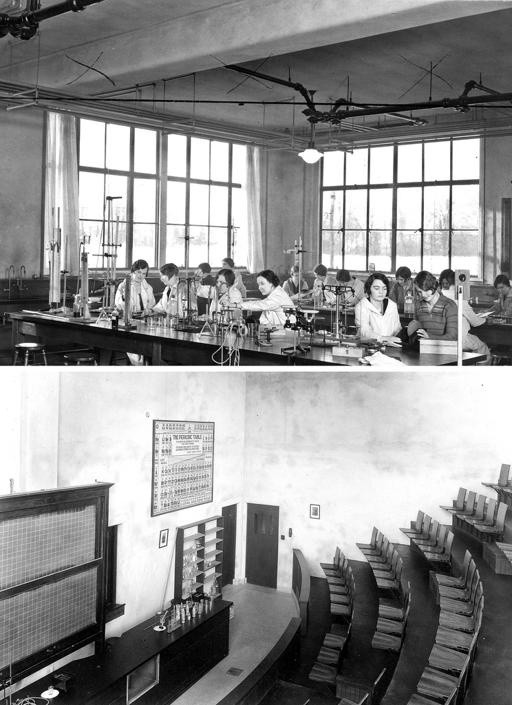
[174,516,225,597]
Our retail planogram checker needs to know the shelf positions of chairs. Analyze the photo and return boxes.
[288,464,512,703]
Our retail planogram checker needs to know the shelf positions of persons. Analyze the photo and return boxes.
[283,266,311,300]
[142,263,189,315]
[355,273,403,349]
[197,263,215,314]
[220,270,296,324]
[441,273,489,361]
[200,269,243,325]
[414,271,458,340]
[389,267,420,312]
[440,269,454,290]
[222,259,247,297]
[487,275,512,318]
[336,269,364,306]
[114,259,161,365]
[312,265,338,303]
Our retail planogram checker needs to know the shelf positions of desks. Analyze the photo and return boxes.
[43,307,486,366]
[241,294,259,302]
[295,291,512,358]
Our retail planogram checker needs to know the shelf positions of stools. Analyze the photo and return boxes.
[12,343,41,362]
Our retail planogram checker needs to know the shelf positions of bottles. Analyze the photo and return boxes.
[167,294,180,329]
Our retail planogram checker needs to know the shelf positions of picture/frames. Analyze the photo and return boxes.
[158,528,168,549]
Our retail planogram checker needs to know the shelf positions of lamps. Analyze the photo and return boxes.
[295,115,323,167]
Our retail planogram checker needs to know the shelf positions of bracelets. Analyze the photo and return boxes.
[234,303,238,309]
